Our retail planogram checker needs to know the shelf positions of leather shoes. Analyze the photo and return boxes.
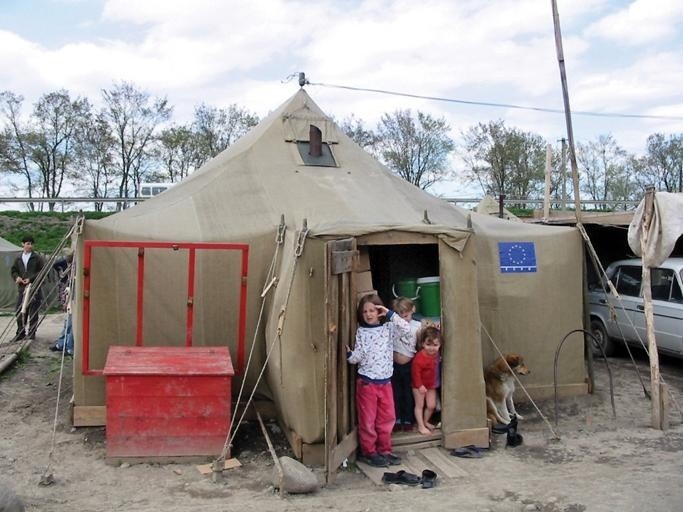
[9,332,36,343]
[50,344,72,356]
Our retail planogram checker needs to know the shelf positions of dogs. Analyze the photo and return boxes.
[483,352,531,425]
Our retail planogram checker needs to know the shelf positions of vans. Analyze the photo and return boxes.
[138,183,175,202]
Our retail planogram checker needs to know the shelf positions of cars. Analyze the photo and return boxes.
[588,257,683,359]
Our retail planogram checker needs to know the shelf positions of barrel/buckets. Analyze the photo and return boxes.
[393,273,418,316]
[415,275,440,316]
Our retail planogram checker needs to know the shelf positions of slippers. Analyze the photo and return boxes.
[381,470,421,485]
[450,445,488,458]
[421,469,437,488]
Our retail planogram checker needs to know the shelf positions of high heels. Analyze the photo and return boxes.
[492,414,523,446]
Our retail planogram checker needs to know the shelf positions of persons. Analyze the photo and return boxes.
[389,295,421,434]
[50,246,74,355]
[10,236,45,342]
[345,293,412,467]
[411,326,443,436]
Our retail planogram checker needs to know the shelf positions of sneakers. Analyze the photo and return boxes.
[356,450,401,467]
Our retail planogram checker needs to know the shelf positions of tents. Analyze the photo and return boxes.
[0,235,58,315]
[74,86,589,482]
